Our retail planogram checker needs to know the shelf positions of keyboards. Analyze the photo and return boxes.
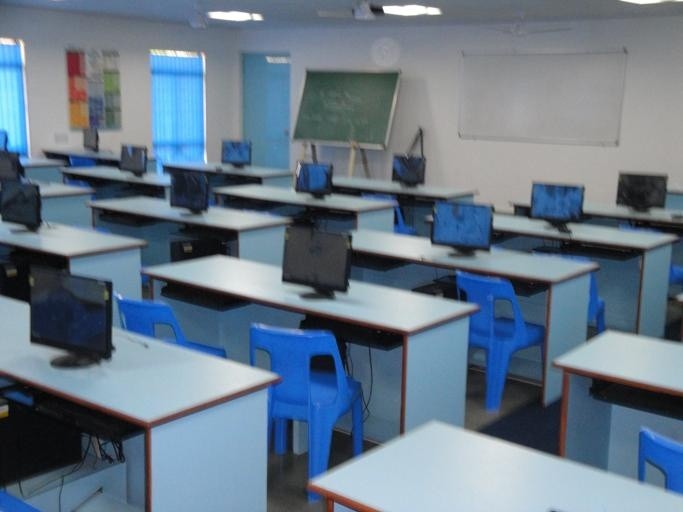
[34,391,141,444]
[299,316,403,350]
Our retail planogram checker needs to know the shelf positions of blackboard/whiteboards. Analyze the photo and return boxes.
[293,68,401,151]
[458,48,628,147]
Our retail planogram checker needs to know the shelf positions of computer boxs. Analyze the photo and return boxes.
[0,395,82,486]
[169,238,213,261]
[1,260,68,302]
[411,275,466,302]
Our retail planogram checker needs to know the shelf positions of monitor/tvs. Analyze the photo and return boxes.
[0,150,20,181]
[615,172,667,213]
[429,200,493,259]
[392,155,426,188]
[0,177,42,234]
[281,225,352,298]
[530,182,585,232]
[29,267,113,366]
[120,144,148,177]
[221,140,251,168]
[295,162,333,198]
[171,172,210,216]
[83,128,99,155]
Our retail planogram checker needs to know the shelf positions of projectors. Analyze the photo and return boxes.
[349,5,383,22]
